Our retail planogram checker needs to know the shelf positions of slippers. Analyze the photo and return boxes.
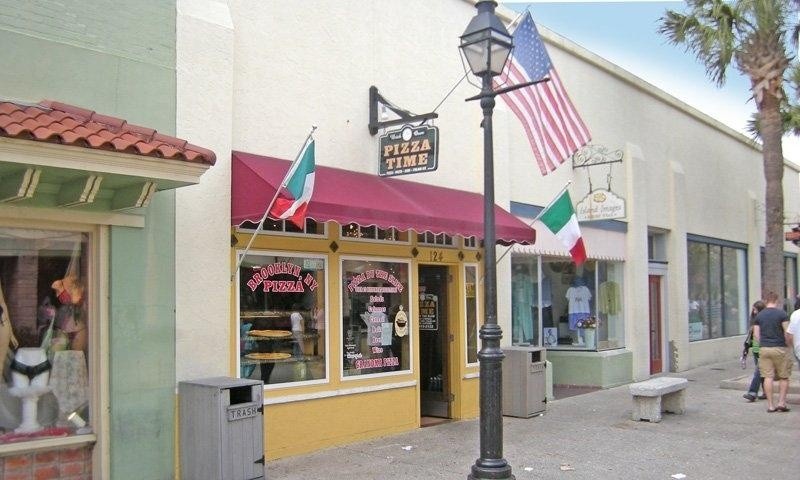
[767,404,790,412]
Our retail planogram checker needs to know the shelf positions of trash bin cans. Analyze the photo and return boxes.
[501,348,547,419]
[180,376,266,480]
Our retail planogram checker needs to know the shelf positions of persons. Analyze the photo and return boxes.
[0,283,19,376]
[240,311,259,379]
[12,347,49,386]
[50,267,87,351]
[742,300,767,401]
[289,306,305,358]
[754,291,792,413]
[785,296,799,370]
[248,311,276,384]
[548,328,552,335]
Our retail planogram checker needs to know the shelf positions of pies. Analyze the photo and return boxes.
[246,353,291,360]
[246,329,292,337]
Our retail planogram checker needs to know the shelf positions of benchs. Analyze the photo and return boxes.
[628,375,688,422]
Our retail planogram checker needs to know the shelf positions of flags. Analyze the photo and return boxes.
[538,192,589,268]
[267,138,316,230]
[486,13,592,180]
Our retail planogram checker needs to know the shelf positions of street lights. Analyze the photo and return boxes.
[458,0,553,479]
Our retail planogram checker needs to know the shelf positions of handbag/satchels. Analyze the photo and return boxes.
[744,330,753,348]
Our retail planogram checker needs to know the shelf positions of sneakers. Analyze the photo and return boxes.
[743,393,755,402]
[758,394,767,399]
